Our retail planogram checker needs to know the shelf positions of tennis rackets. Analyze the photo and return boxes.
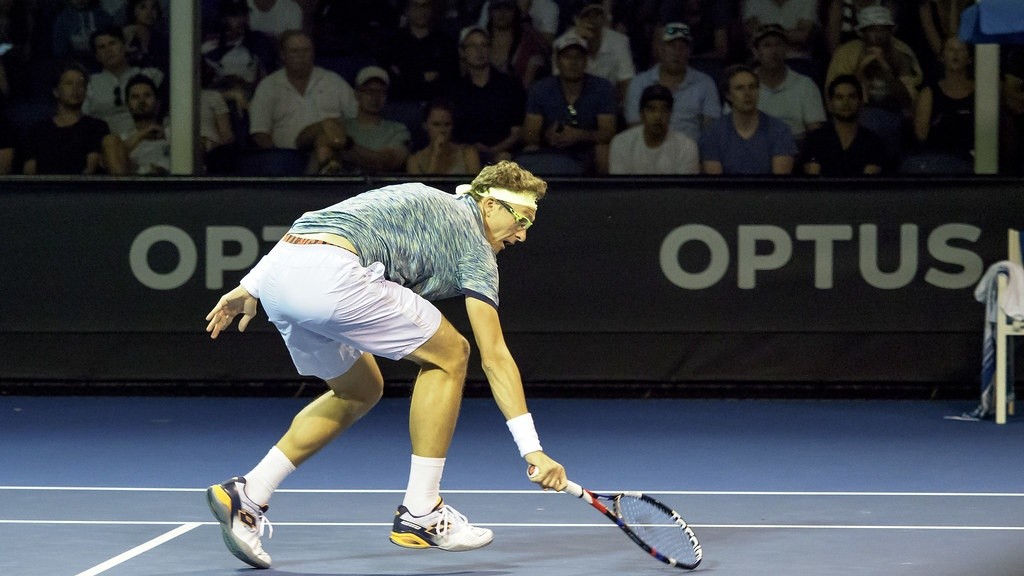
[526,464,704,569]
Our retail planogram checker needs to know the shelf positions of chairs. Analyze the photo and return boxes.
[993,229,1024,425]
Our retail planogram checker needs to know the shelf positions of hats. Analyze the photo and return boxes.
[550,31,588,52]
[460,25,492,43]
[659,21,695,43]
[355,65,392,86]
[851,5,898,29]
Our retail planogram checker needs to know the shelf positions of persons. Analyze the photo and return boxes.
[205,160,569,570]
[0,0,1023,180]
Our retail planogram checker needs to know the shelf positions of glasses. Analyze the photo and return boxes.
[497,199,533,230]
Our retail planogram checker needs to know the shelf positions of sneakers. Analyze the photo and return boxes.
[207,477,273,569]
[389,497,495,551]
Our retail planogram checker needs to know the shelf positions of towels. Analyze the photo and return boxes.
[974,259,1024,320]
